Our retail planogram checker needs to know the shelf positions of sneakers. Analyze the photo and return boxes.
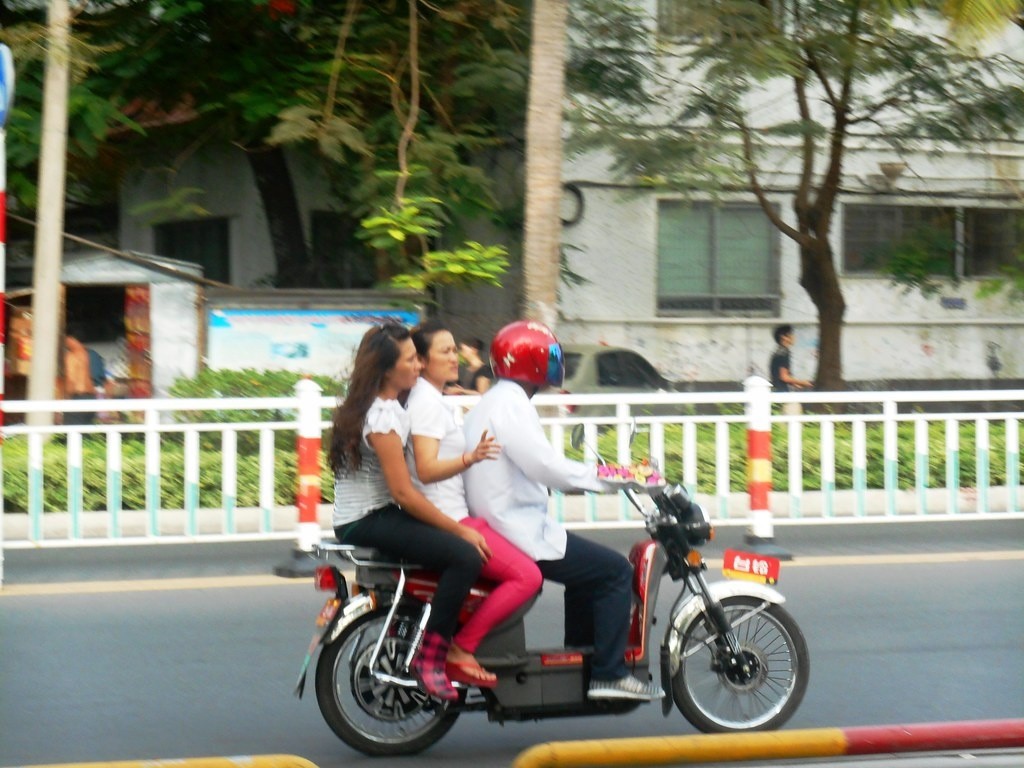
[587,676,667,700]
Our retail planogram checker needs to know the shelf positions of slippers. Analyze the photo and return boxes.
[443,654,498,687]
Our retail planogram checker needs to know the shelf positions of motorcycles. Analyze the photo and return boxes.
[294,419,812,758]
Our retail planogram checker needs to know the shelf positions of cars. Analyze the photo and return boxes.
[536,347,687,422]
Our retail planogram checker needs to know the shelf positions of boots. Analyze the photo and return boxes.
[408,633,458,700]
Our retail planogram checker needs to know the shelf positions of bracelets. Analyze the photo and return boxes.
[462,452,469,467]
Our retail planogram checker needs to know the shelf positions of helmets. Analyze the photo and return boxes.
[490,321,564,389]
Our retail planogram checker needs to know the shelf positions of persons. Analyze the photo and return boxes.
[459,319,669,699]
[61,329,107,445]
[398,321,543,684]
[770,325,813,391]
[333,325,492,701]
[442,336,493,396]
